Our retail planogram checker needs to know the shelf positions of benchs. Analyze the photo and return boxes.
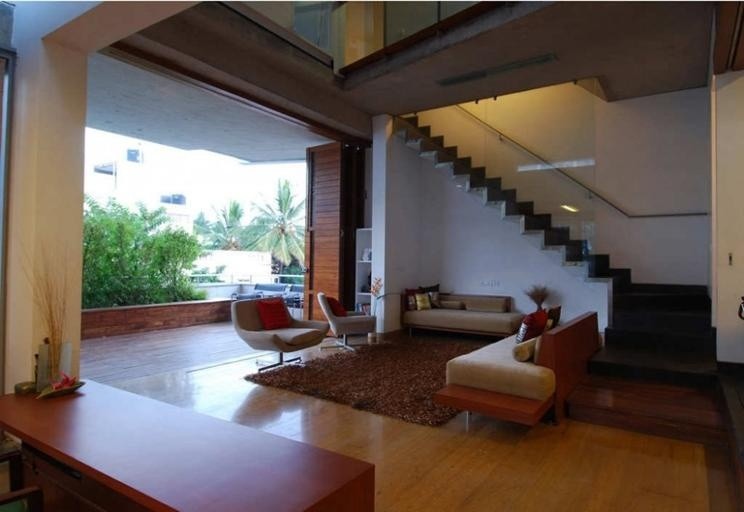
[253,283,304,308]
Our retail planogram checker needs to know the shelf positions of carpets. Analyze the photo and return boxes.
[244,330,499,431]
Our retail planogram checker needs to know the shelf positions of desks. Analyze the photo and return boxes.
[1,377,377,512]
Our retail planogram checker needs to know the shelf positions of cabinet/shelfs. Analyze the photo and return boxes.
[354,228,371,316]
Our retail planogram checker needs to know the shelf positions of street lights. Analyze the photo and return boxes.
[93,157,118,198]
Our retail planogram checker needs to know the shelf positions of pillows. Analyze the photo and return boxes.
[326,297,348,318]
[510,336,537,363]
[254,298,294,331]
[545,305,562,331]
[404,284,441,312]
[515,307,548,345]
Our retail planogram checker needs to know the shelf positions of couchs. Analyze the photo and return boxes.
[436,310,600,428]
[399,292,526,339]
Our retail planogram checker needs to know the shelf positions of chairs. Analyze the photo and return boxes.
[318,292,377,354]
[230,295,331,373]
[0,450,45,512]
